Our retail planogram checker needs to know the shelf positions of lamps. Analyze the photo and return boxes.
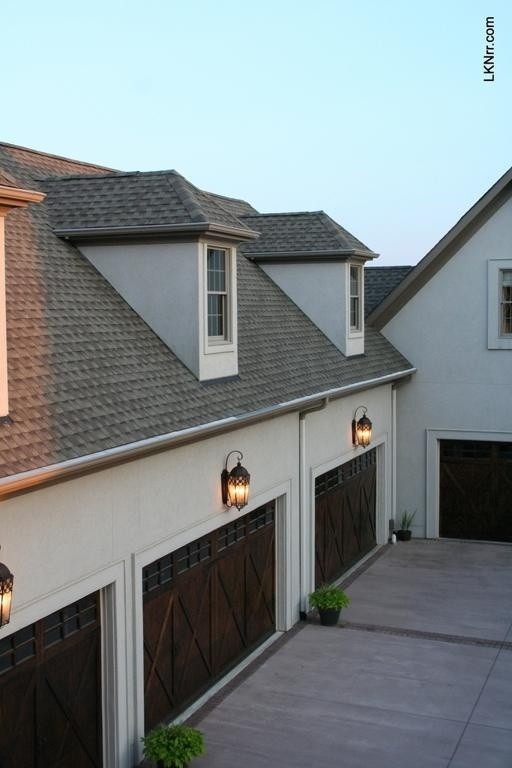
[0,545,14,627]
[351,403,374,451]
[219,448,253,512]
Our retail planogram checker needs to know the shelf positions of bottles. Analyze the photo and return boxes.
[391,531,397,544]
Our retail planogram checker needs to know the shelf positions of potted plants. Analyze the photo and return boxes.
[308,581,349,627]
[395,508,417,543]
[139,721,207,768]
